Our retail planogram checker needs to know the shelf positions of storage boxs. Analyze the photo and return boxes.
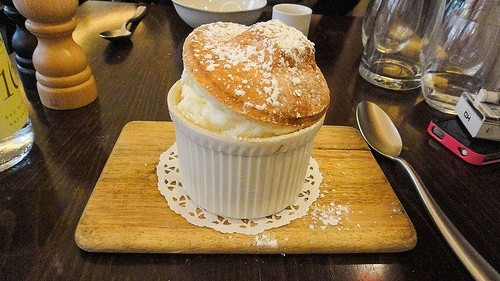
[454,91,500,141]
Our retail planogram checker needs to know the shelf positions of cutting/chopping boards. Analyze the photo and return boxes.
[73,117,418,254]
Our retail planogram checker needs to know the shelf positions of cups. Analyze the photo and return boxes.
[271,3,313,39]
[358,0,500,117]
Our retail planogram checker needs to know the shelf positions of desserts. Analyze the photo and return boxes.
[168,17,330,221]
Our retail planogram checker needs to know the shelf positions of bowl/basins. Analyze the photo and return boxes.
[165,79,326,218]
[171,0,268,29]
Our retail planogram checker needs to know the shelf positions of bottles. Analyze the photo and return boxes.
[0,30,35,173]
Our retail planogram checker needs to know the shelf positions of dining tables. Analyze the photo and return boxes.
[0,0,500,281]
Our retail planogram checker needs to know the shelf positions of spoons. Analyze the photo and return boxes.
[354,99,500,281]
[98,5,147,40]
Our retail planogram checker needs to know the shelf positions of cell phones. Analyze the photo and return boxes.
[428,116,499,166]
